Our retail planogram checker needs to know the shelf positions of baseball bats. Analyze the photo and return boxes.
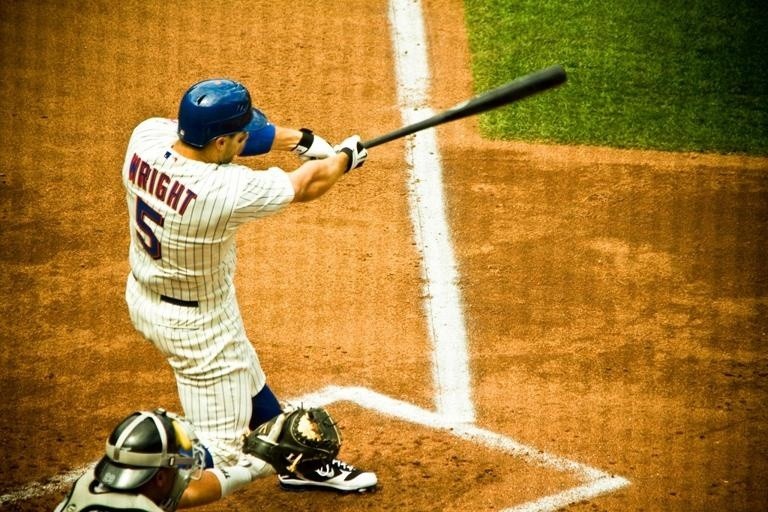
[309,65,566,160]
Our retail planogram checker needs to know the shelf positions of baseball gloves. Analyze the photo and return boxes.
[241,406,339,476]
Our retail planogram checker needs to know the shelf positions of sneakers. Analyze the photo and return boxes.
[279,458,377,497]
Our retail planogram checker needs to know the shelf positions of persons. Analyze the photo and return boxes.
[124,78,380,510]
[51,408,207,512]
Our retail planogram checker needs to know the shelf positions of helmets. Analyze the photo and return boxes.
[95,409,205,511]
[176,78,269,149]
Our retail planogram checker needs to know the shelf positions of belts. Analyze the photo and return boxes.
[159,293,198,307]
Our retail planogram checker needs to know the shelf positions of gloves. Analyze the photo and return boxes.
[340,136,367,172]
[291,127,337,159]
